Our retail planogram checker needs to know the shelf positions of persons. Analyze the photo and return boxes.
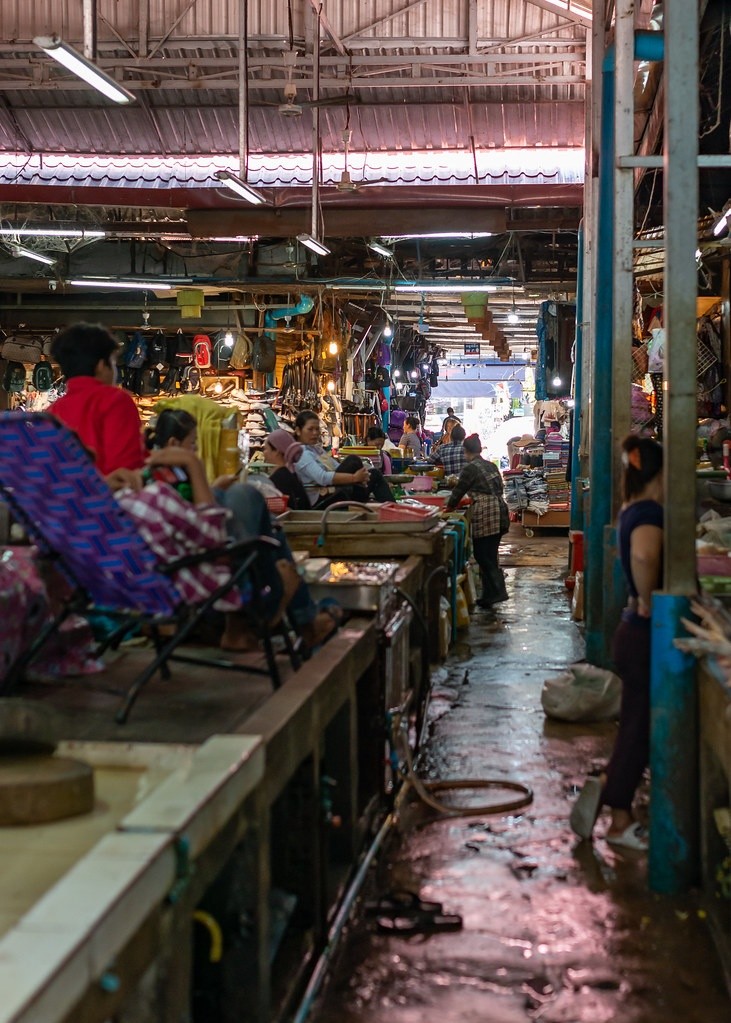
[364,427,393,475]
[39,322,153,475]
[293,411,397,511]
[260,429,313,511]
[431,407,471,475]
[569,433,665,854]
[399,417,423,458]
[445,433,510,610]
[98,448,344,650]
[144,409,241,502]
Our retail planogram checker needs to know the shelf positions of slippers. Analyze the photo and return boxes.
[605,822,649,849]
[363,890,444,921]
[376,909,463,934]
[571,778,603,837]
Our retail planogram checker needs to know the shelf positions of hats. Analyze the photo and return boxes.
[514,433,540,447]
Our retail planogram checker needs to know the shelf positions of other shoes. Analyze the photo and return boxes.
[476,594,510,607]
[345,406,374,416]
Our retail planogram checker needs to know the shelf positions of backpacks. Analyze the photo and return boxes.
[364,345,392,390]
[110,322,277,398]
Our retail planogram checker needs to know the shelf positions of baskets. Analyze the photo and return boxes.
[264,497,290,513]
[377,503,438,520]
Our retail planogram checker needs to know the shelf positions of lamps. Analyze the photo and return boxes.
[215,168,266,207]
[32,34,137,107]
[366,242,394,258]
[12,246,56,266]
[297,234,331,258]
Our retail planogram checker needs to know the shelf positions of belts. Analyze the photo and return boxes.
[277,355,321,413]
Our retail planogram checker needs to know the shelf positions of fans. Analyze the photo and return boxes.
[293,129,388,196]
[266,292,321,333]
[131,290,167,333]
[258,236,313,276]
[220,48,357,117]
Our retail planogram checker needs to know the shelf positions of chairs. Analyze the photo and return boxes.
[0,412,300,714]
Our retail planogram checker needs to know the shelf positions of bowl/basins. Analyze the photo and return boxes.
[401,496,446,507]
[401,476,437,491]
[706,480,731,502]
[338,446,380,470]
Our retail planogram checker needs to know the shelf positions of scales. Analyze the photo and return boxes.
[384,475,414,500]
[408,464,436,476]
[248,463,275,478]
